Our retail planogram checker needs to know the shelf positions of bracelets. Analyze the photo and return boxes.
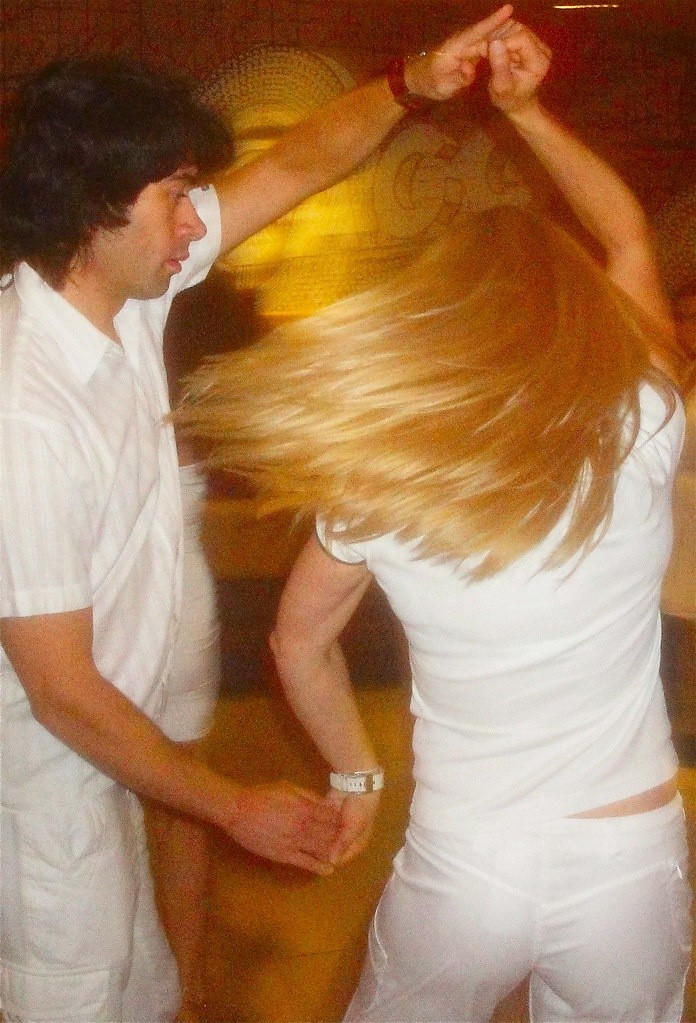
[329,766,385,795]
[386,52,431,112]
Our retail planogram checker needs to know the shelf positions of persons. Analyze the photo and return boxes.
[0,4,696,1023]
